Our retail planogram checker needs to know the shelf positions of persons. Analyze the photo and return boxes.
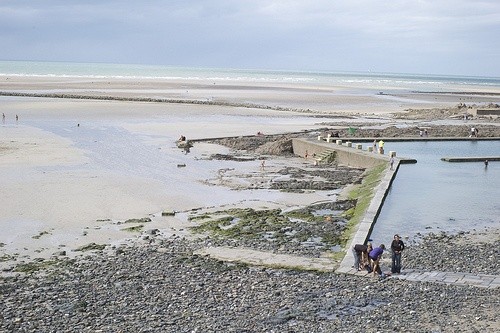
[378,140,384,154]
[369,244,385,278]
[15,114,18,120]
[391,234,405,274]
[470,126,478,138]
[389,155,394,170]
[179,135,185,141]
[2,113,5,118]
[372,139,376,153]
[425,129,428,137]
[305,150,308,161]
[419,129,423,137]
[312,152,316,157]
[260,160,265,171]
[352,244,372,271]
